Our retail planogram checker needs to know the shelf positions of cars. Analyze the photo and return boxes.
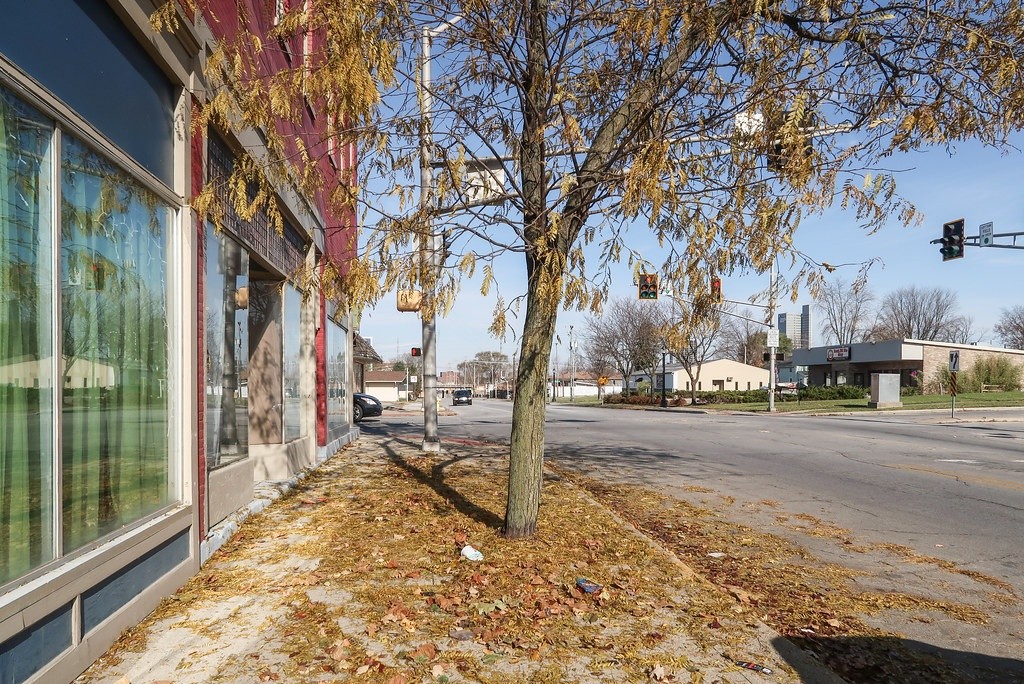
[353,393,382,422]
[453,390,473,406]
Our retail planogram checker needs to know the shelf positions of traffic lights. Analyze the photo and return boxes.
[939,218,964,261]
[639,273,658,298]
[709,277,722,303]
